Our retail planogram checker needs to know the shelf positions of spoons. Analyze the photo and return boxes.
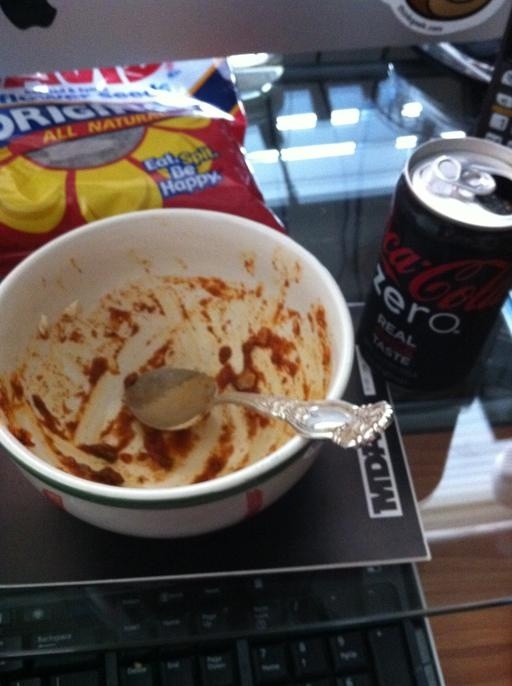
[129,365,393,447]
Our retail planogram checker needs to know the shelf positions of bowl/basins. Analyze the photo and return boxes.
[2,210,356,539]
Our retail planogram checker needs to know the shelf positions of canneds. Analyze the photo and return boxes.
[355,136,512,399]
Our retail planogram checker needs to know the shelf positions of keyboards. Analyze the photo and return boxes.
[0,562,448,686]
